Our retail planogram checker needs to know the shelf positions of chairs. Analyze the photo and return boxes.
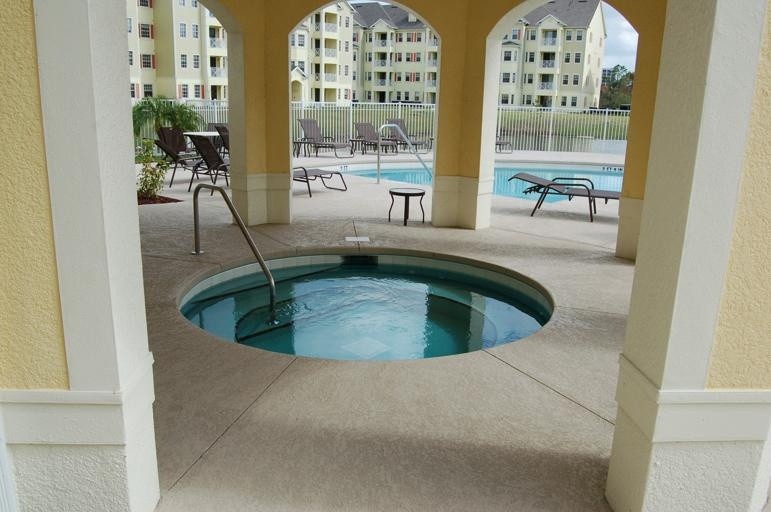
[153,123,230,197]
[293,166,347,198]
[496,135,513,154]
[507,172,621,223]
[293,118,430,159]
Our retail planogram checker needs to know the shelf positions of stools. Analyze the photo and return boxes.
[388,188,425,226]
